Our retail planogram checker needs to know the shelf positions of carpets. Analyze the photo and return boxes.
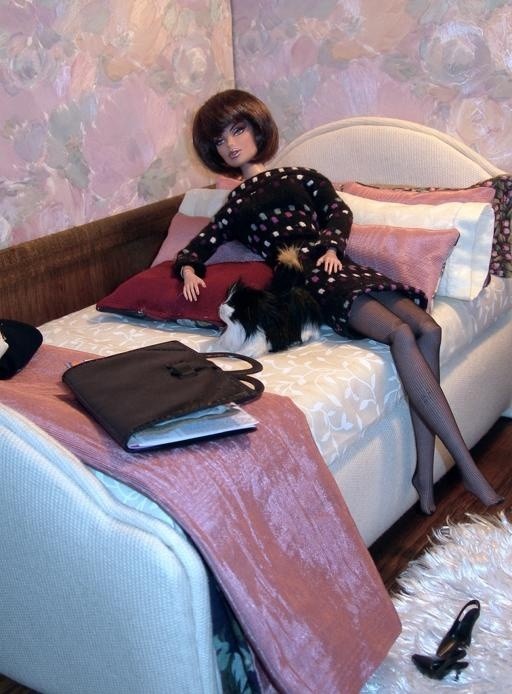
[354,508,512,694]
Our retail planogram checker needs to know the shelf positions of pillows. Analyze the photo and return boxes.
[97,173,512,332]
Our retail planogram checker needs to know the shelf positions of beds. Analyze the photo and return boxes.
[0,115,512,694]
